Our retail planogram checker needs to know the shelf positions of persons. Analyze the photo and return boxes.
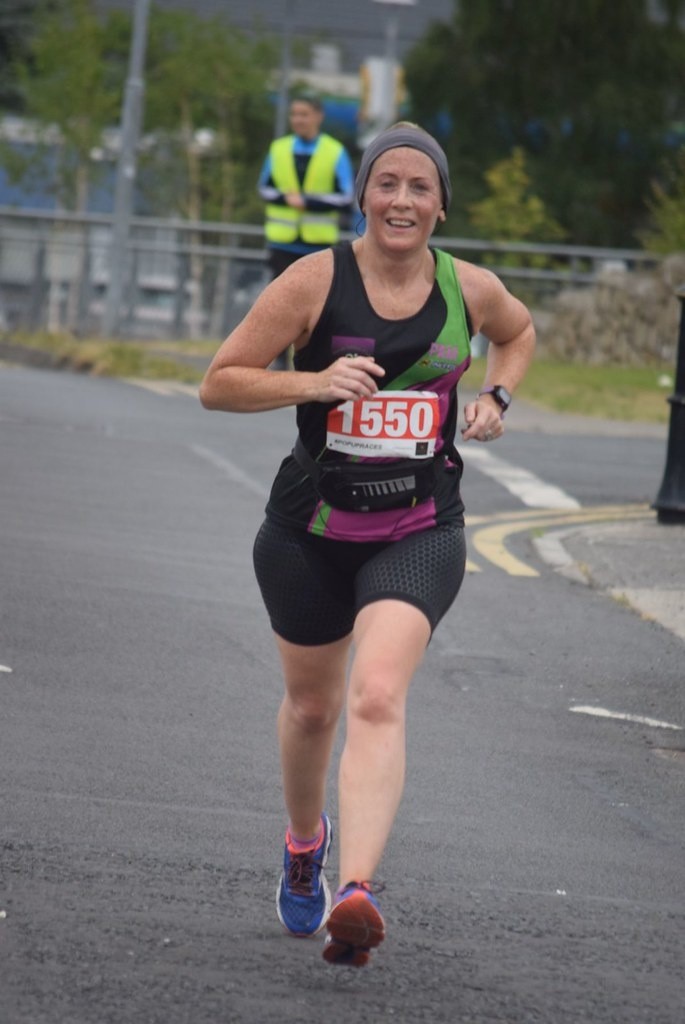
[199,121,535,967]
[257,92,353,370]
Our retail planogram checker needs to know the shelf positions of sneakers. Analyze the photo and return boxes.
[275,813,333,938]
[322,881,387,972]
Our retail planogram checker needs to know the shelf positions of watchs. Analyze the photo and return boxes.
[478,384,511,421]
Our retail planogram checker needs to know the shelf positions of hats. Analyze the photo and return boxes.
[355,121,451,218]
